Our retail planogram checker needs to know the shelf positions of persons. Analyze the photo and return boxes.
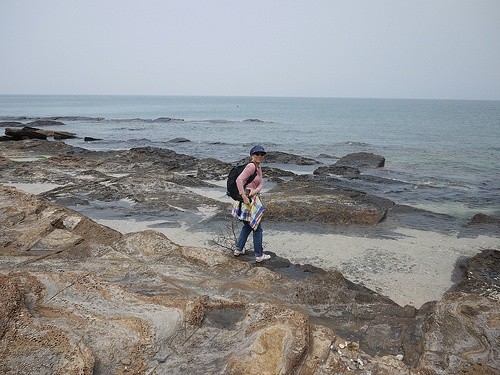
[231,145,271,262]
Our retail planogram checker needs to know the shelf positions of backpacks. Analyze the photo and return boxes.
[226,164,247,201]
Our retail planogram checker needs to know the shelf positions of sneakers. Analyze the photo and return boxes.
[255,253,271,262]
[234,247,245,256]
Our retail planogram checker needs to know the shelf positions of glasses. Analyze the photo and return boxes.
[253,153,265,156]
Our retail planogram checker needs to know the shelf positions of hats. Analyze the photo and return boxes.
[250,145,266,156]
